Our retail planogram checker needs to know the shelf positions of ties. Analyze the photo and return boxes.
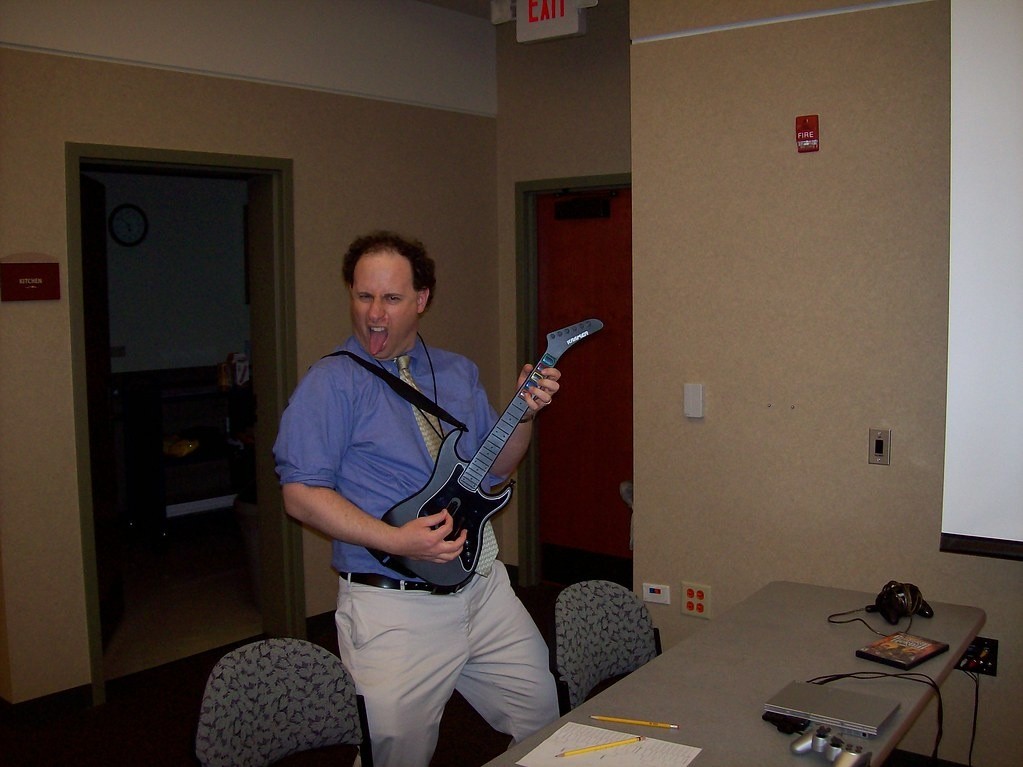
[394,355,500,578]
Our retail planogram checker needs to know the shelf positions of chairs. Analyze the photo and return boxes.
[556,580,663,718]
[195,639,375,767]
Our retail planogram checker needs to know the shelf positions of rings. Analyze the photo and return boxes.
[541,398,552,405]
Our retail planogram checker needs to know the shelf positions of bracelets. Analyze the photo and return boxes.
[519,412,535,423]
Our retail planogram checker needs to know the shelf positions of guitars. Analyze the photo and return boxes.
[377,318,606,588]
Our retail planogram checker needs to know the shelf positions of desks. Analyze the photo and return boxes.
[480,580,987,767]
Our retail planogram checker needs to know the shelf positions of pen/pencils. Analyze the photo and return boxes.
[590,715,676,730]
[556,738,643,759]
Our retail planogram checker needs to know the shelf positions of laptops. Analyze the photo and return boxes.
[764,679,901,740]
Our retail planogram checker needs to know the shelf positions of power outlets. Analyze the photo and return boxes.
[953,637,1000,677]
[868,427,891,466]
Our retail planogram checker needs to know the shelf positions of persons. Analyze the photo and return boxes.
[271,230,560,767]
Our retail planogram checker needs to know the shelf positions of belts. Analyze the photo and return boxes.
[338,568,476,595]
